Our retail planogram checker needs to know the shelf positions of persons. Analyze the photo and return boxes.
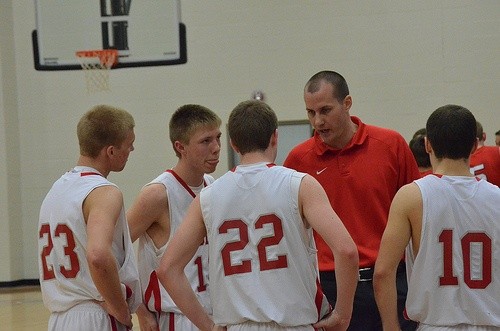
[157,99,358,331]
[469,122,500,187]
[373,105,500,331]
[283,71,421,331]
[38,105,133,331]
[125,103,222,331]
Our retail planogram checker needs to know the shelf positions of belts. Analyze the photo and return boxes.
[324,260,406,284]
